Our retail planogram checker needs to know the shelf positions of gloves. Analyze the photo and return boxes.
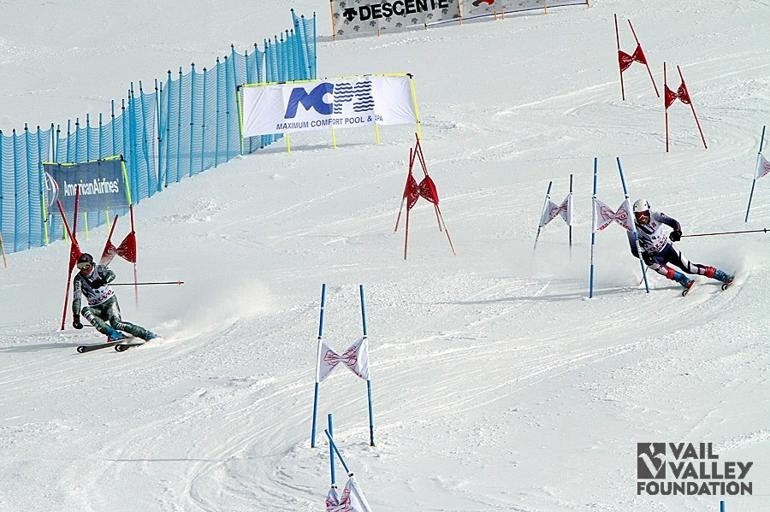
[89,279,106,289]
[642,252,656,266]
[71,312,84,330]
[668,230,683,242]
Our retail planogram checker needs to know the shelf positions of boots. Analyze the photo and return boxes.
[712,268,733,283]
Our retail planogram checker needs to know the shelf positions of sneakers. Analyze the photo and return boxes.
[105,328,125,342]
[143,330,161,344]
[678,275,692,288]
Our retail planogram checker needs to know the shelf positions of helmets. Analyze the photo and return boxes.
[75,252,94,271]
[631,197,651,213]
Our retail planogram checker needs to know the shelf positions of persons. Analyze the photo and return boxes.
[71,253,159,343]
[626,200,735,292]
